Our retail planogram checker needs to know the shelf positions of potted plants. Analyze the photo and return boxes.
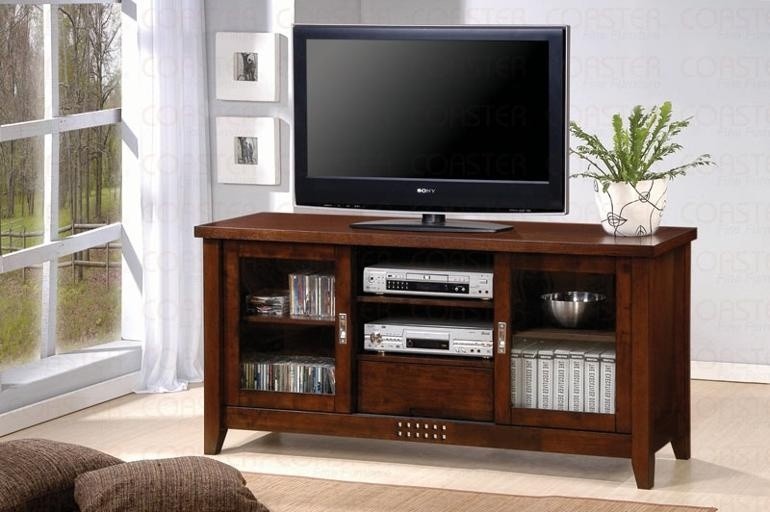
[567,101,720,238]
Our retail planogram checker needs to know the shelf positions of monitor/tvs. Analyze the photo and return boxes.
[288,23,570,235]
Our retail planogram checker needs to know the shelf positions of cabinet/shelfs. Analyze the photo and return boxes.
[194,212,697,490]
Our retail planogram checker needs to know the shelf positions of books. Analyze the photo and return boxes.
[510,337,617,415]
[240,353,336,395]
[244,273,335,322]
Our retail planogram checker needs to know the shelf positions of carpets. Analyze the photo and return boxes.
[241,470,720,512]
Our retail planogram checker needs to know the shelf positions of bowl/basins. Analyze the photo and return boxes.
[538,291,609,328]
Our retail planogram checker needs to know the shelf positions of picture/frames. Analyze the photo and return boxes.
[214,31,281,186]
